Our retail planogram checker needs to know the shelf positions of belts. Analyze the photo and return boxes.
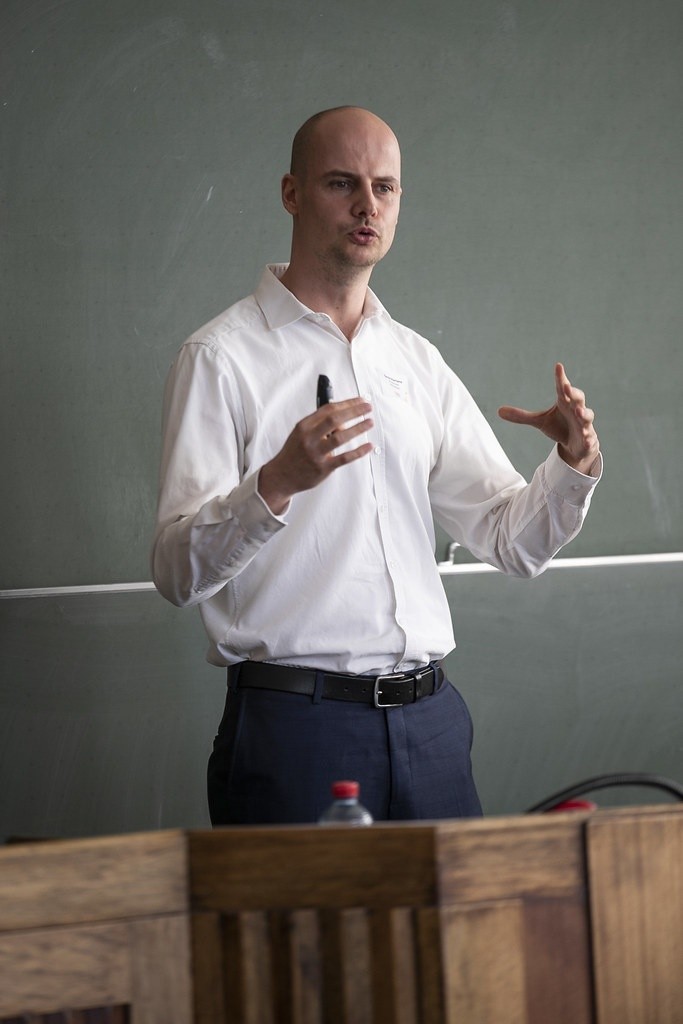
[227,660,442,707]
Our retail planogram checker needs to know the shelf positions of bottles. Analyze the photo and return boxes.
[317,780,375,821]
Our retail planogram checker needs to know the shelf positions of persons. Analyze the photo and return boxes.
[145,104,605,828]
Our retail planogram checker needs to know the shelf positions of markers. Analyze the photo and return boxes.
[316,373,338,440]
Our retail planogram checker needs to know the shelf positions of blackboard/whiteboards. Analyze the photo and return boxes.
[0,1,683,847]
